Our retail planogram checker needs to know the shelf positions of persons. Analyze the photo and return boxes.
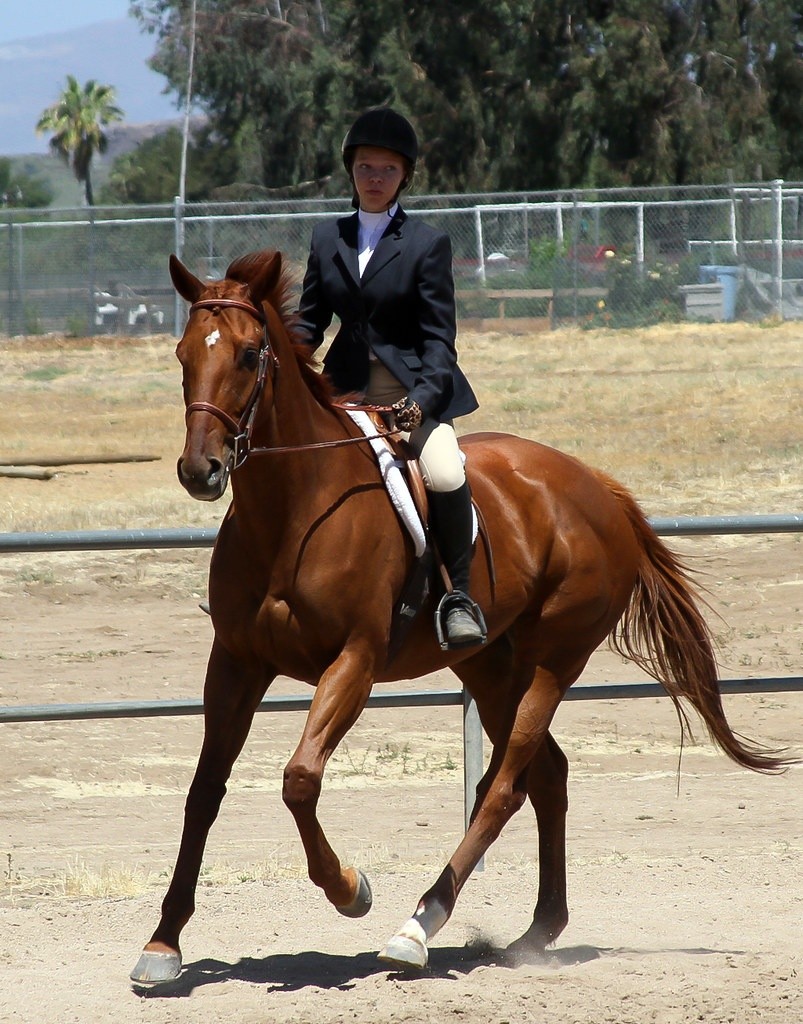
[287,106,484,643]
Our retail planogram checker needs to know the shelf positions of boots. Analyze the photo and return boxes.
[425,479,482,643]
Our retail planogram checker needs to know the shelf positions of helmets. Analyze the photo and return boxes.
[341,108,417,187]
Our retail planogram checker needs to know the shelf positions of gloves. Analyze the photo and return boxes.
[392,397,422,431]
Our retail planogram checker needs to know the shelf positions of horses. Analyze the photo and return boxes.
[129,247,801,985]
